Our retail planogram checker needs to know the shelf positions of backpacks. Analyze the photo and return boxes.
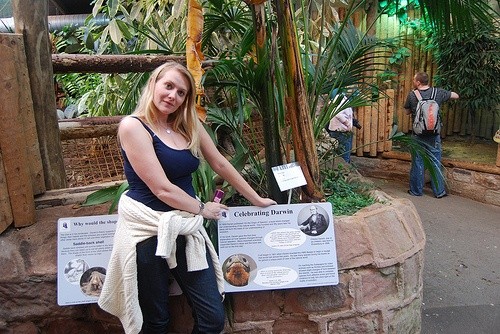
[413,86,442,138]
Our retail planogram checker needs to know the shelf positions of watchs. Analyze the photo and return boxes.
[196,201,204,215]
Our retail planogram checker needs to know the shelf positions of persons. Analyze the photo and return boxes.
[299,205,328,236]
[403,72,460,199]
[327,93,355,164]
[116,60,279,334]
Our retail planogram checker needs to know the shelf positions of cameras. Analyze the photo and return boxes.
[353,119,362,129]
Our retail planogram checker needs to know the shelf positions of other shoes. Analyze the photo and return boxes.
[436,194,449,199]
[408,189,423,197]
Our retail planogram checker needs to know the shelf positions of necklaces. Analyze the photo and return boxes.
[158,121,170,134]
